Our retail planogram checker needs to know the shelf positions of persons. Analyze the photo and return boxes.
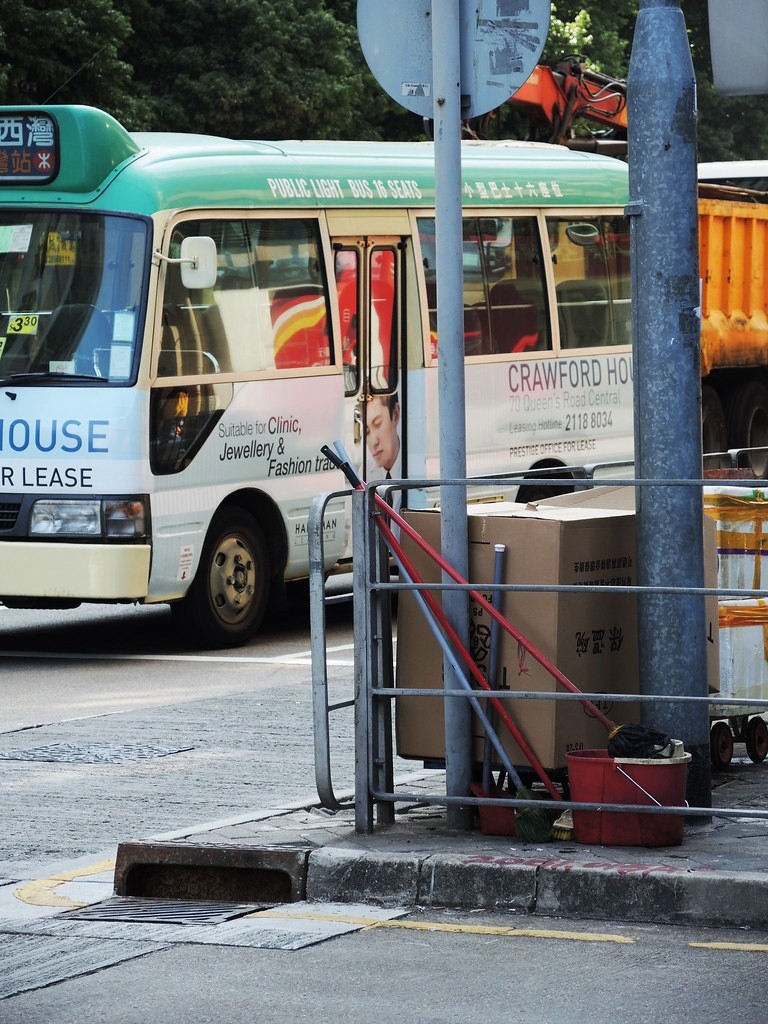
[353,392,409,553]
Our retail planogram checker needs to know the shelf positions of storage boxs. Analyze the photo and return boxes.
[703,485,768,600]
[397,502,638,772]
[707,599,768,717]
[706,513,719,696]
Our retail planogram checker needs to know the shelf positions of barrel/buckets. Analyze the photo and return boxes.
[565,738,693,847]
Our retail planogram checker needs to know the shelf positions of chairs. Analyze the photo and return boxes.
[486,280,547,353]
[556,279,610,353]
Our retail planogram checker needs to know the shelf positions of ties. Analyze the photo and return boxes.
[383,473,394,530]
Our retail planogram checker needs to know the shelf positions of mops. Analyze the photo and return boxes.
[317,431,700,762]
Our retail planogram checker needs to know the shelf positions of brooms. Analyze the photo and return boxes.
[318,438,584,841]
